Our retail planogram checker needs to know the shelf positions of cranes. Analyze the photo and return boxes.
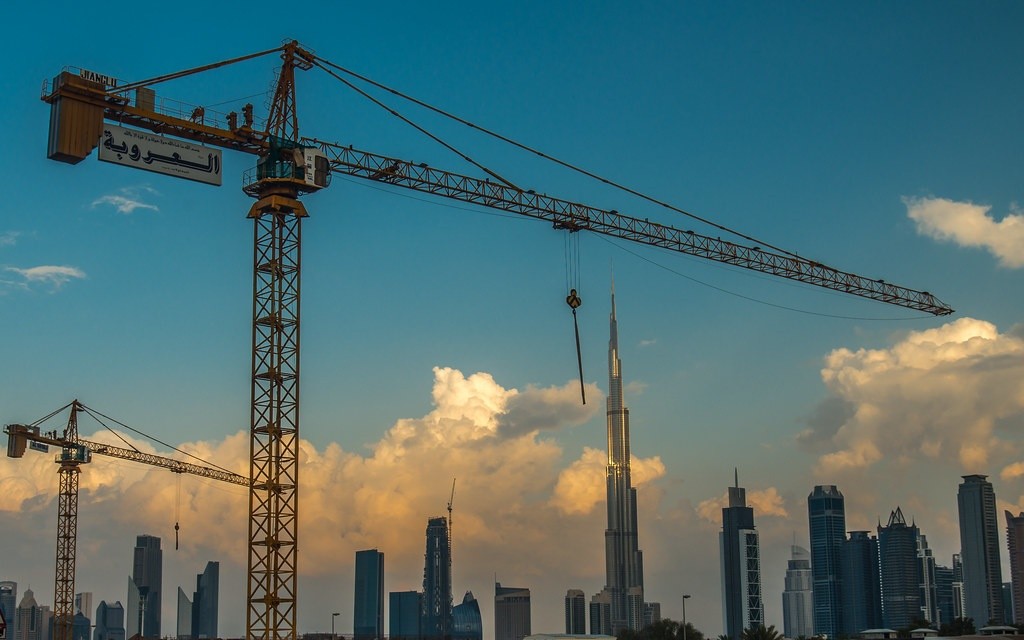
[4,396,286,640]
[39,33,953,640]
[447,478,457,558]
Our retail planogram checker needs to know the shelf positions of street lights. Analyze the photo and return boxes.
[682,594,691,640]
[332,612,340,640]
